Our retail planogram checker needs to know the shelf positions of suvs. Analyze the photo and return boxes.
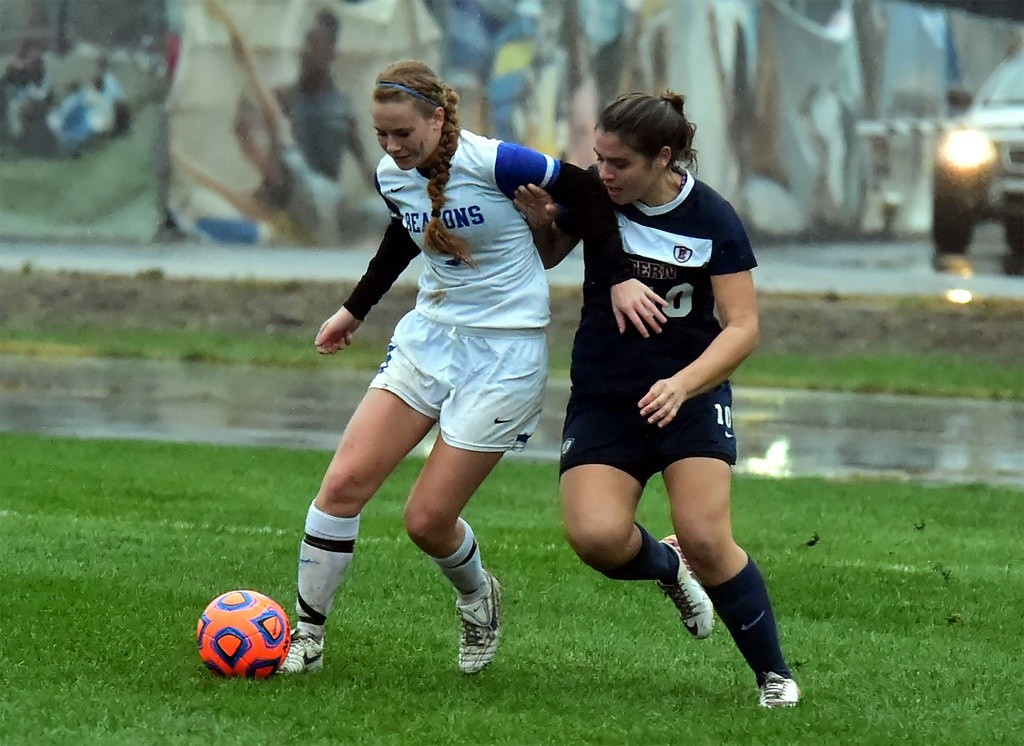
[933,39,1024,271]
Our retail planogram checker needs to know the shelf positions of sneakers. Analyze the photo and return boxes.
[276,627,325,674]
[758,671,801,710]
[454,569,503,676]
[653,534,715,639]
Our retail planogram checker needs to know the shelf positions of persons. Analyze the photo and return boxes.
[514,91,801,710]
[0,38,132,156]
[711,0,884,235]
[420,1,687,163]
[278,59,669,674]
[235,7,367,208]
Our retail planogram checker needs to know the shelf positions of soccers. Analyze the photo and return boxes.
[195,589,291,680]
